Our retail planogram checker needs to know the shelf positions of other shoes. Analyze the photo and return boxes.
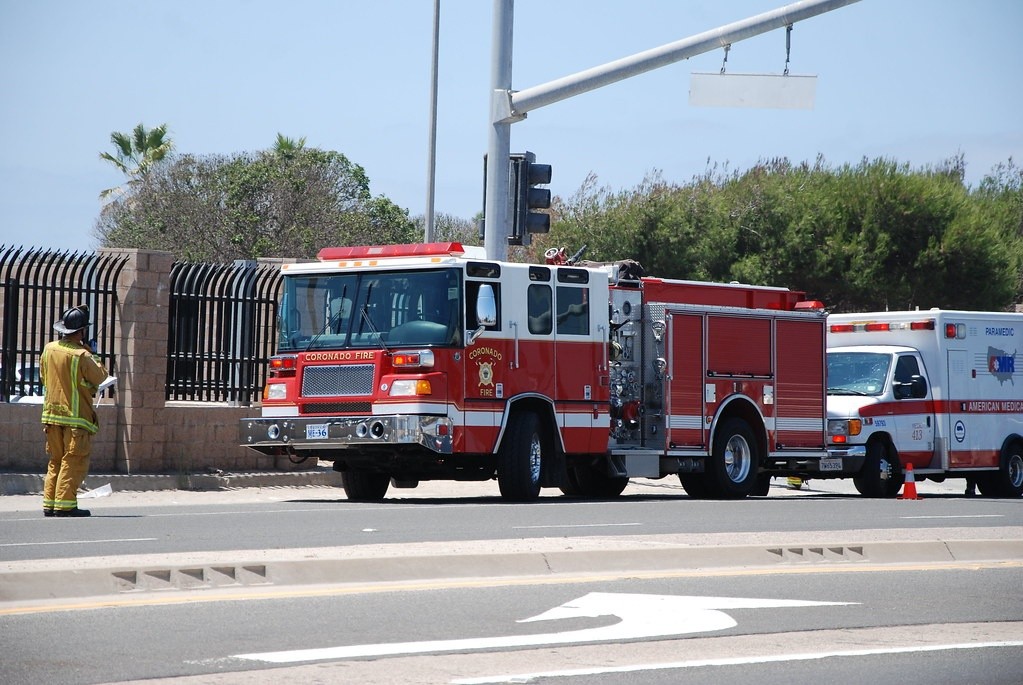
[44,509,54,516]
[53,507,90,517]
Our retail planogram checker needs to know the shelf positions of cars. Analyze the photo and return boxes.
[0,363,42,396]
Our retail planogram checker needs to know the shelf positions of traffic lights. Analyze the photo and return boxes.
[516,152,553,247]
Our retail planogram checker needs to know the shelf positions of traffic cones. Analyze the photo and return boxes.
[896,462,922,501]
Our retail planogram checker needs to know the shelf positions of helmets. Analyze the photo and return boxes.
[53,304,91,334]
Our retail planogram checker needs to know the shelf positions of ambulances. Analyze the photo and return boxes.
[826,305,1023,499]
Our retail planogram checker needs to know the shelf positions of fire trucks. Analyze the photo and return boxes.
[239,242,829,504]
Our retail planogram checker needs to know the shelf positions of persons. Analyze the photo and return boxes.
[39,304,108,517]
